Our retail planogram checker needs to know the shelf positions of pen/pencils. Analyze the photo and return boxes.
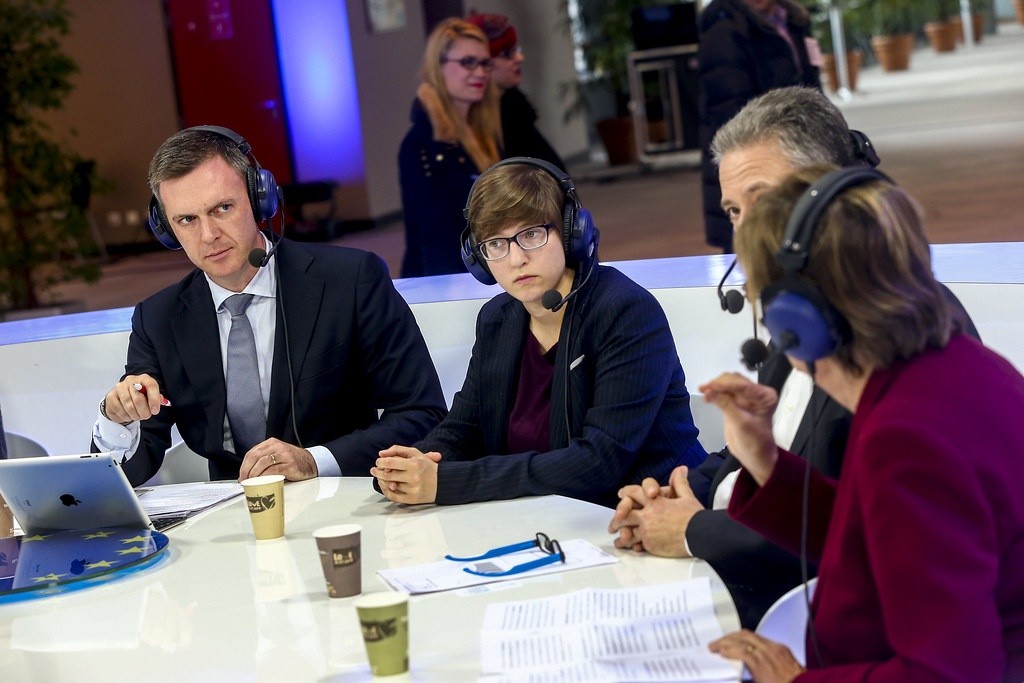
[132,383,174,407]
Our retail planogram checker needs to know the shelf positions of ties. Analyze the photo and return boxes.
[217,293,267,456]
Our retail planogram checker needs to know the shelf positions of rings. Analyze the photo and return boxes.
[746,645,755,654]
[270,454,276,464]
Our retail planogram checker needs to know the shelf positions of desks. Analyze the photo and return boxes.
[0,474,744,683]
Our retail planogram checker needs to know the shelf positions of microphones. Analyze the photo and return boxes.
[542,227,600,312]
[718,257,744,314]
[741,331,798,370]
[249,186,284,267]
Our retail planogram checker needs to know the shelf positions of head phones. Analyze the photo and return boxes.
[460,156,596,285]
[846,128,880,169]
[761,165,898,361]
[148,124,279,249]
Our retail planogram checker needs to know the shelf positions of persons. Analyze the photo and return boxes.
[607,87,981,632]
[693,0,823,253]
[91,125,450,482]
[399,15,500,280]
[699,164,1024,683]
[370,156,709,509]
[466,13,568,174]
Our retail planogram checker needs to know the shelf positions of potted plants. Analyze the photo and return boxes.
[865,0,915,72]
[954,0,984,43]
[810,0,862,92]
[569,0,642,167]
[921,0,956,53]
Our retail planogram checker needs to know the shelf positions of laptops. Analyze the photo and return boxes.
[13,529,152,589]
[0,451,187,533]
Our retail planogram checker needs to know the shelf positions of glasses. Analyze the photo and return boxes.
[496,45,526,60]
[444,533,565,578]
[443,55,495,74]
[474,220,557,262]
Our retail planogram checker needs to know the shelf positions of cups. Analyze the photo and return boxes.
[356,591,409,677]
[313,524,364,598]
[242,475,286,541]
